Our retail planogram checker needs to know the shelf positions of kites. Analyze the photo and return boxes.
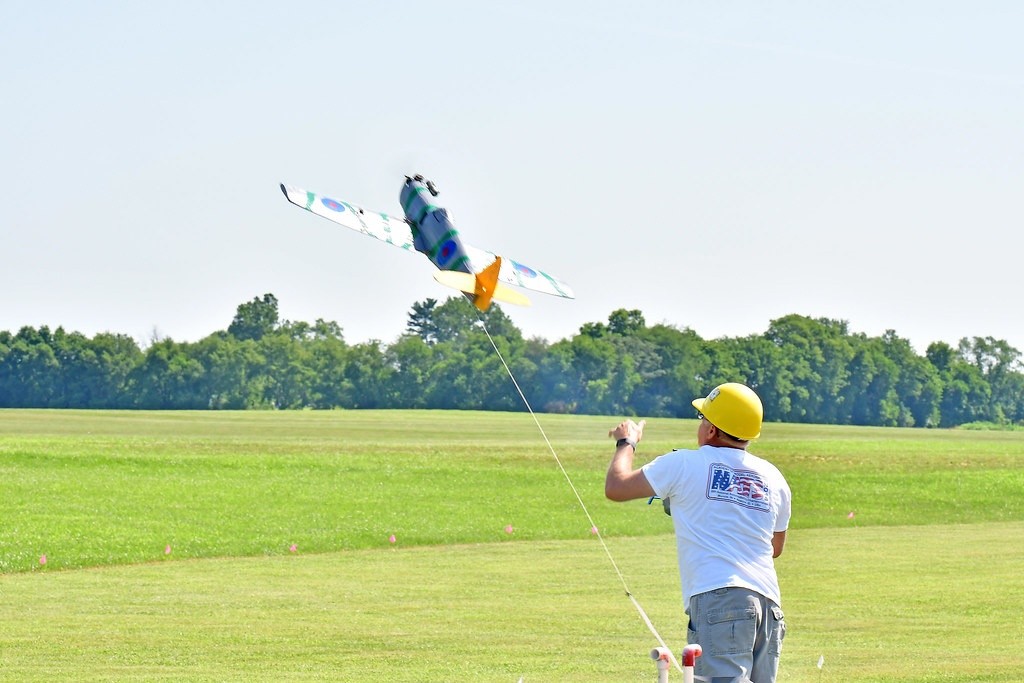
[279,172,577,314]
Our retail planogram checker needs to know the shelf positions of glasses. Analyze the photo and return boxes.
[697,412,710,422]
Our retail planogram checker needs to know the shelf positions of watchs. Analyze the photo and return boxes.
[616,438,637,453]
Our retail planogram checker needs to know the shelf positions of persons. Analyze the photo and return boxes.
[605,383,792,683]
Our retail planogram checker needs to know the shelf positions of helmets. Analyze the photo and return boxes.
[692,383,762,439]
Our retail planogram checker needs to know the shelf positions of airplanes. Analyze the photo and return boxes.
[279,167,578,312]
